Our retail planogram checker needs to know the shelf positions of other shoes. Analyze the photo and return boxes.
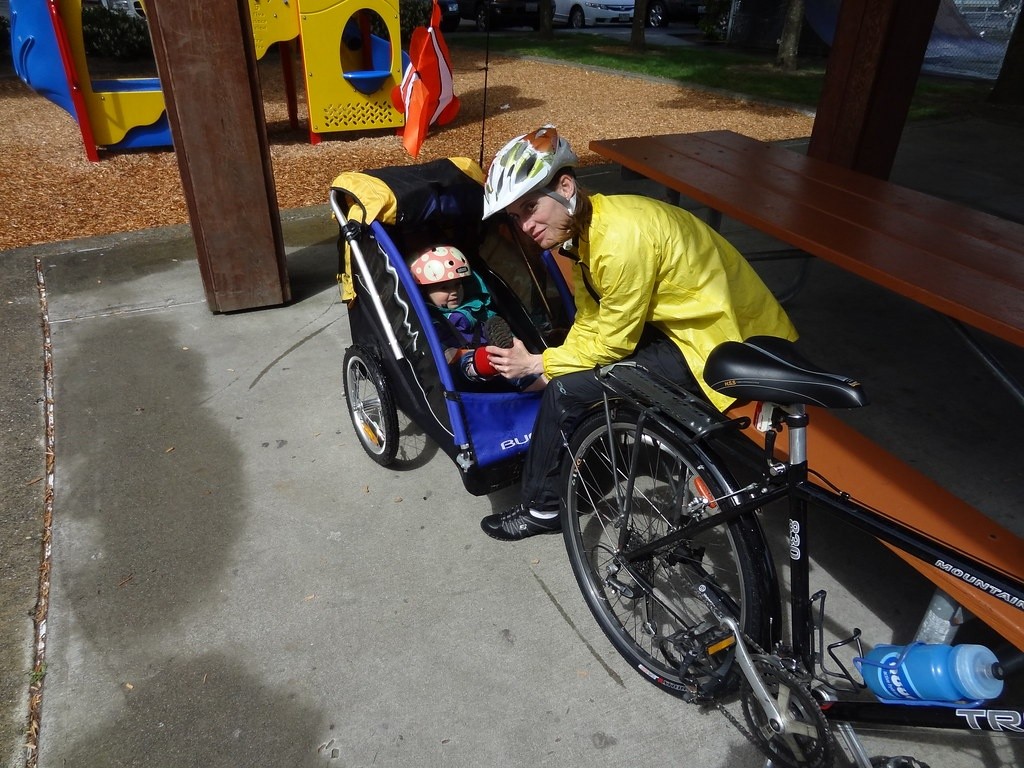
[482,315,517,354]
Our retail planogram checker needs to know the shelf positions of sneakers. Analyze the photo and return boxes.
[480,502,565,543]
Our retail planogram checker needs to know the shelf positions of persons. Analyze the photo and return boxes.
[482,123,798,542]
[410,240,548,394]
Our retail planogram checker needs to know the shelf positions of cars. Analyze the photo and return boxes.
[646,0,722,29]
[555,0,634,29]
[438,0,556,31]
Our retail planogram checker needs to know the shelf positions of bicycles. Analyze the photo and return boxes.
[552,336,1024,768]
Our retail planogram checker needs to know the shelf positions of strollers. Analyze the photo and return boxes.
[324,153,581,498]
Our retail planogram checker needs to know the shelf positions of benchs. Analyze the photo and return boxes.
[552,247,1024,658]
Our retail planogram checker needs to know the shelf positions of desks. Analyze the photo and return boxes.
[589,130,1024,417]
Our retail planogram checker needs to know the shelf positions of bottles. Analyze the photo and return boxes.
[862,644,1006,700]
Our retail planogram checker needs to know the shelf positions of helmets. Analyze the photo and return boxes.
[481,124,579,221]
[407,242,472,285]
